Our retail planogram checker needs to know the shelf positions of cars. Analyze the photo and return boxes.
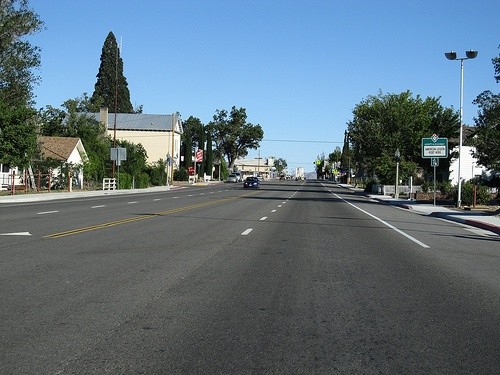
[226,174,238,183]
[244,177,259,188]
[231,172,242,182]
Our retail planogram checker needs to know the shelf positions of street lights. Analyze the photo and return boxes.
[444,50,479,208]
[395,149,400,199]
